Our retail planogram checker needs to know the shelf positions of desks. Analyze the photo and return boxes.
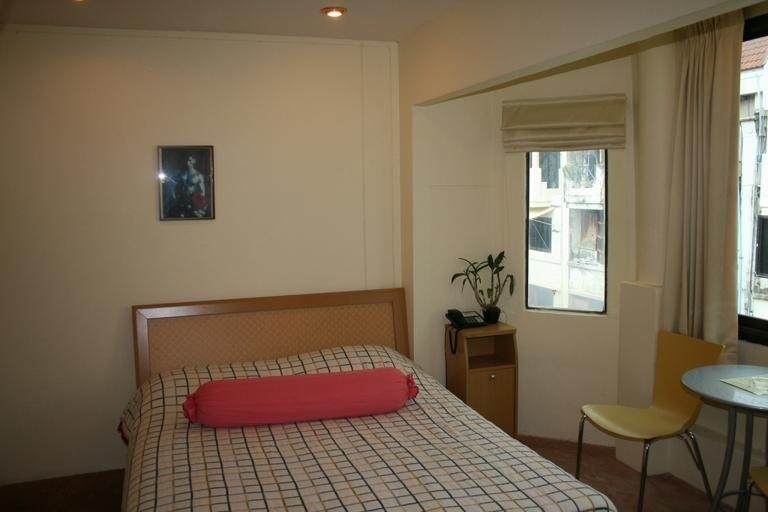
[680,364,768,512]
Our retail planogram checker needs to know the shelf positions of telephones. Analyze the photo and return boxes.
[445,309,487,329]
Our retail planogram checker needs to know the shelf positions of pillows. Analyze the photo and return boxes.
[181,367,419,428]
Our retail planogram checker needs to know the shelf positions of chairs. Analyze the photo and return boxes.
[748,467,768,512]
[576,332,726,512]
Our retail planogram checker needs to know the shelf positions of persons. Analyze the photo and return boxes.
[171,154,206,218]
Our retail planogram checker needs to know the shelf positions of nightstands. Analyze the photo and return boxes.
[444,321,518,437]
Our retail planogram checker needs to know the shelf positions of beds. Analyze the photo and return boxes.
[121,287,617,512]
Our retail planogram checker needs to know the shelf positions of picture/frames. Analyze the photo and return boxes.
[157,144,215,221]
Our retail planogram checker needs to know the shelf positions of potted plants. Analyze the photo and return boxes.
[450,250,514,323]
